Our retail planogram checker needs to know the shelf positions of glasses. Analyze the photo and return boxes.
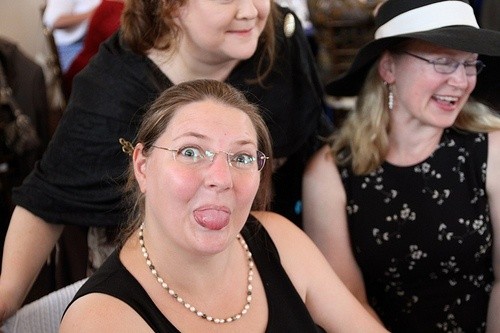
[400,48,487,76]
[150,141,268,174]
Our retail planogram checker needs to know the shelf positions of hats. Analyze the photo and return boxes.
[324,0,500,98]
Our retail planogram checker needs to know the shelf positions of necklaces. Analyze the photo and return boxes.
[139,219,255,323]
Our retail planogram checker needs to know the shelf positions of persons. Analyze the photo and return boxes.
[2,0,333,317]
[44,0,103,71]
[303,0,500,332]
[56,80,393,333]
